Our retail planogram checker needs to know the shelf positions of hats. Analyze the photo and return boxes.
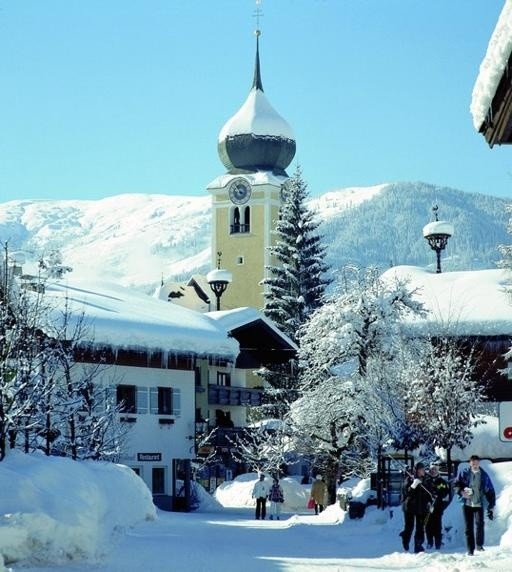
[430,462,439,472]
[316,475,322,480]
[261,474,265,478]
[416,463,424,470]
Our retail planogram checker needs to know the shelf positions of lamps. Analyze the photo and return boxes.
[188,434,201,441]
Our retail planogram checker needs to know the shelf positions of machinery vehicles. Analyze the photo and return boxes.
[348,453,415,520]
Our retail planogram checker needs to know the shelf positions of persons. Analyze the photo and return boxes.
[252,474,270,519]
[312,473,326,516]
[399,463,432,554]
[425,461,451,549]
[269,478,284,520]
[455,453,494,554]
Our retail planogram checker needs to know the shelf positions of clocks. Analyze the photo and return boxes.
[228,180,251,206]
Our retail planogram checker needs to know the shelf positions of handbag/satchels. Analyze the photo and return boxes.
[308,500,315,508]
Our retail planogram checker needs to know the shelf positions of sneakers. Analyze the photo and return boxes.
[269,515,273,520]
[277,516,280,520]
[414,543,425,552]
[468,550,473,555]
[477,545,484,551]
[435,543,441,549]
[401,536,409,551]
[426,543,432,549]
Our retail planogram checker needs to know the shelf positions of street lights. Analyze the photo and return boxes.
[204,409,211,495]
[203,250,233,311]
[422,201,454,274]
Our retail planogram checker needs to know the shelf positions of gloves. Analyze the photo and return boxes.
[280,499,283,503]
[269,497,271,500]
[411,478,422,489]
[429,506,434,513]
